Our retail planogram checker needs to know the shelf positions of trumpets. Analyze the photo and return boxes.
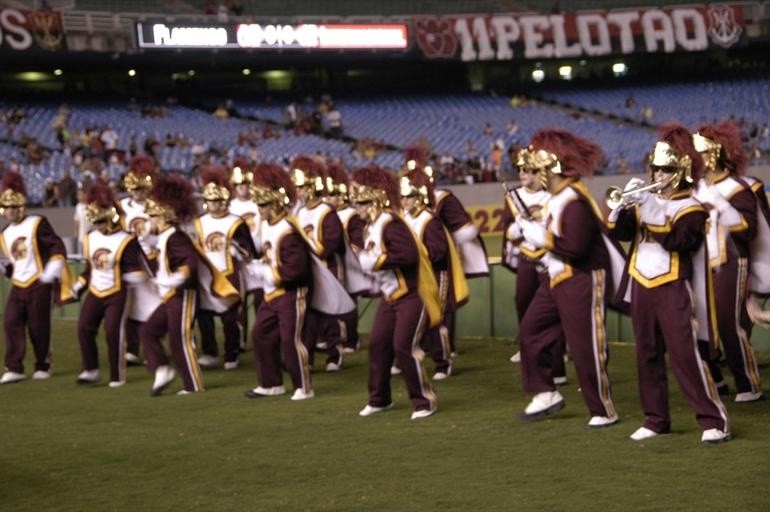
[604,181,662,210]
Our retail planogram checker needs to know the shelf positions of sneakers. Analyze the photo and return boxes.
[108,380,126,388]
[585,411,619,429]
[551,367,766,405]
[123,352,140,363]
[701,428,731,444]
[244,385,285,397]
[630,426,672,441]
[291,387,314,400]
[509,351,521,362]
[32,369,53,380]
[410,403,438,419]
[223,357,240,370]
[432,365,453,379]
[0,372,27,384]
[390,366,402,375]
[178,389,205,395]
[518,390,565,421]
[325,355,343,371]
[315,342,328,350]
[359,401,395,417]
[151,365,176,396]
[197,355,219,365]
[77,369,101,384]
[342,341,360,352]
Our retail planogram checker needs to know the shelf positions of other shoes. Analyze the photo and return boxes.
[450,351,456,357]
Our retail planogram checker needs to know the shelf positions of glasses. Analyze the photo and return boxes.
[649,165,679,173]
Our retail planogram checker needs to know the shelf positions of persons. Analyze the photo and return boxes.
[601,123,732,443]
[0,87,770,428]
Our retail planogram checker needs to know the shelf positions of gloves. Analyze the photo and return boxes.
[246,259,275,287]
[515,213,548,251]
[454,225,480,242]
[702,185,741,228]
[608,177,646,223]
[151,272,186,289]
[358,250,379,272]
[39,259,64,283]
[122,273,147,284]
[627,190,667,226]
[70,282,83,300]
[507,221,523,240]
